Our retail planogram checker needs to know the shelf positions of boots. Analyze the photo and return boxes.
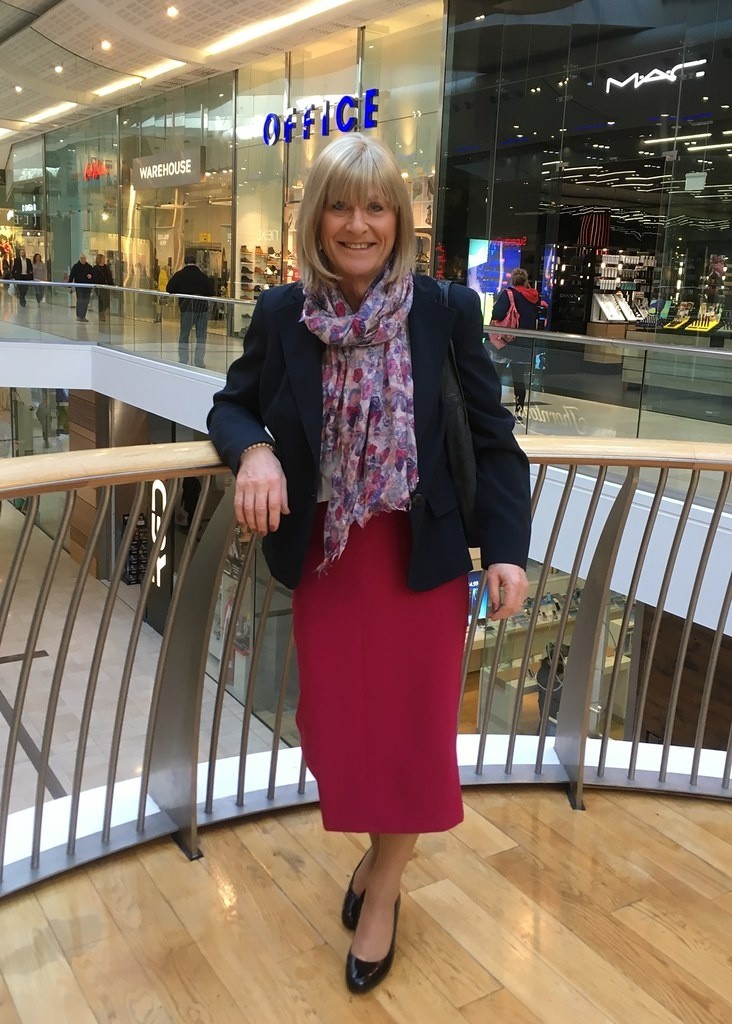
[239,246,300,318]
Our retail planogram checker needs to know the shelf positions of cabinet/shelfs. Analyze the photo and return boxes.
[537,243,732,398]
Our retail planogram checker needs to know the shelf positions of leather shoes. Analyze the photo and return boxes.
[346,887,400,993]
[343,846,374,929]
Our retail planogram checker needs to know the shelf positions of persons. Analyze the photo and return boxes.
[536,650,566,736]
[89,253,118,322]
[64,253,93,324]
[9,249,32,308]
[165,256,211,369]
[202,129,533,998]
[179,476,201,531]
[34,389,70,449]
[491,269,541,416]
[29,253,47,307]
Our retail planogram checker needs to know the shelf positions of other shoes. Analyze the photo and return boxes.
[20,301,25,307]
[37,303,40,307]
[76,317,80,321]
[79,318,89,321]
[99,316,105,321]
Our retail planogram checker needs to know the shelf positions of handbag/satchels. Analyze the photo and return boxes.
[434,280,485,547]
[488,288,519,350]
